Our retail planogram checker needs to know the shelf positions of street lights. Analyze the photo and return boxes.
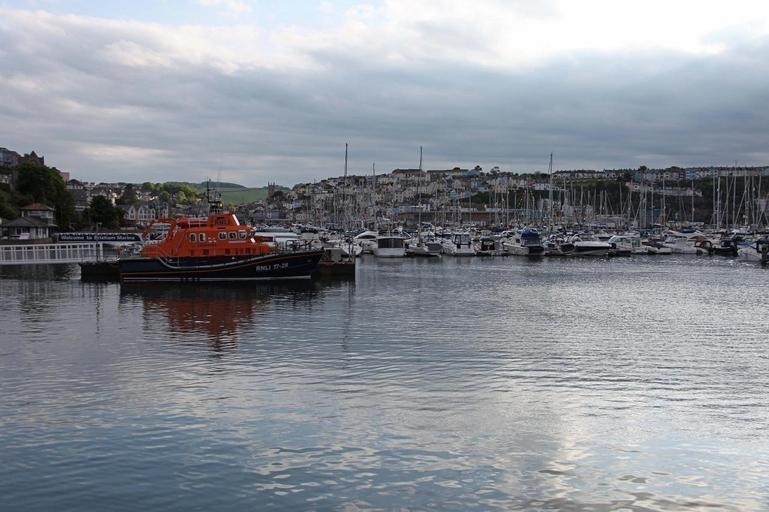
[96,222,102,247]
